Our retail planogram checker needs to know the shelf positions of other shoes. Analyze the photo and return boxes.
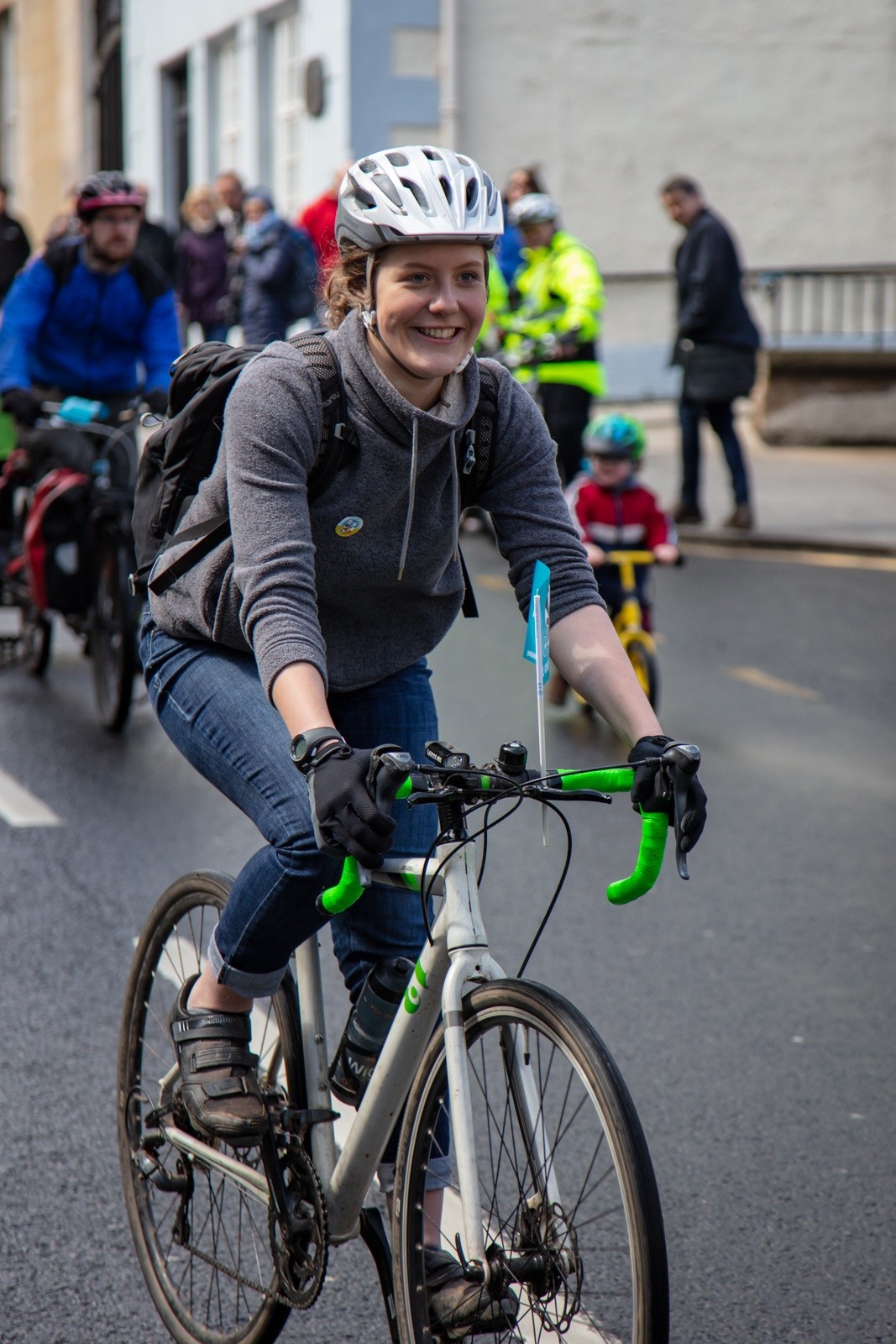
[670,504,700,523]
[725,505,753,528]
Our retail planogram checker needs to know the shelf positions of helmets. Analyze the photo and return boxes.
[75,170,143,219]
[508,192,560,228]
[581,413,644,460]
[334,144,505,251]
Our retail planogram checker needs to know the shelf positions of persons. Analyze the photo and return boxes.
[0,170,179,674]
[134,165,356,346]
[473,160,604,494]
[549,413,682,710]
[135,145,705,1344]
[0,181,80,464]
[659,176,759,532]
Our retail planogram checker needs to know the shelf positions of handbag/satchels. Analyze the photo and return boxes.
[683,347,756,401]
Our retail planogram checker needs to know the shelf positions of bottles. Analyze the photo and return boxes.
[327,958,409,1104]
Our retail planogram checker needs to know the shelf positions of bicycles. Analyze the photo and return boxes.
[2,409,141,733]
[111,737,700,1344]
[567,550,686,717]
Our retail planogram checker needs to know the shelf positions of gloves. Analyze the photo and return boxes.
[2,389,50,429]
[303,740,416,870]
[627,735,708,854]
[141,389,169,414]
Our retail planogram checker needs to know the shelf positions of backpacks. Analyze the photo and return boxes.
[127,328,499,598]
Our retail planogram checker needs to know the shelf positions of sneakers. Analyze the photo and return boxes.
[166,973,269,1147]
[423,1244,519,1339]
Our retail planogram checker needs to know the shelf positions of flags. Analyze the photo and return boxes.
[521,556,555,696]
